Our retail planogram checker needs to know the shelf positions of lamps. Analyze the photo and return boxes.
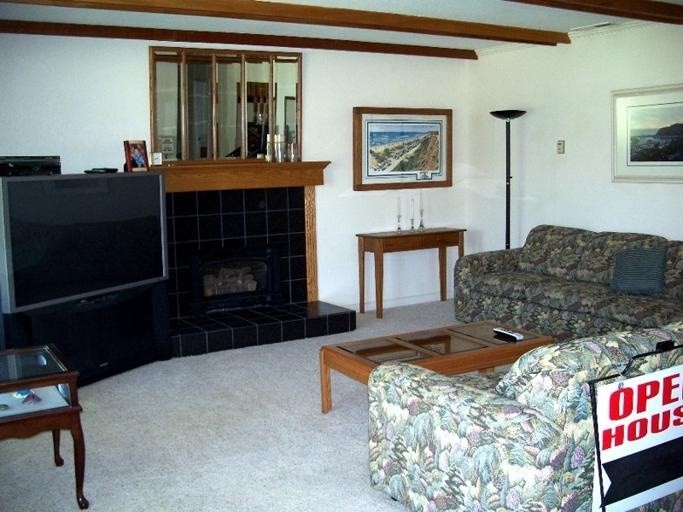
[489,106,531,248]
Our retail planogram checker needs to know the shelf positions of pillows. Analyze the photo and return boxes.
[605,244,668,298]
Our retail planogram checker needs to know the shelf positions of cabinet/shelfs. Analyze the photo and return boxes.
[0,275,178,390]
[0,341,93,512]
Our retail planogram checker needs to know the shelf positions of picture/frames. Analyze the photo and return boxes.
[350,105,456,194]
[123,138,151,174]
[607,80,683,186]
[282,95,295,145]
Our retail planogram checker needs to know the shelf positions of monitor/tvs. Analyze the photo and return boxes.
[0,172,169,314]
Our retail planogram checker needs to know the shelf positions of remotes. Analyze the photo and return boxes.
[93,168,118,172]
[493,327,524,341]
[85,170,106,173]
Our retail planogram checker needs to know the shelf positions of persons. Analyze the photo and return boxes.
[129,146,145,167]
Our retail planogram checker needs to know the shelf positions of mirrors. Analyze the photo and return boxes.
[269,50,302,162]
[182,47,219,160]
[211,46,245,160]
[147,42,186,161]
[242,50,272,161]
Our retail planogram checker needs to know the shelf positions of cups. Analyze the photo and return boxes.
[256,154,263,160]
[287,143,298,162]
[264,155,271,163]
[272,132,286,162]
[266,134,270,156]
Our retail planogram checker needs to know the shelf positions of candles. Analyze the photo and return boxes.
[410,197,414,220]
[397,195,402,217]
[418,192,423,211]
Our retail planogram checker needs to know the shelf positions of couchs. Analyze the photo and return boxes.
[366,317,681,512]
[451,223,682,349]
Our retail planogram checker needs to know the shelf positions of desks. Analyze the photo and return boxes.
[316,319,556,417]
[354,225,468,320]
